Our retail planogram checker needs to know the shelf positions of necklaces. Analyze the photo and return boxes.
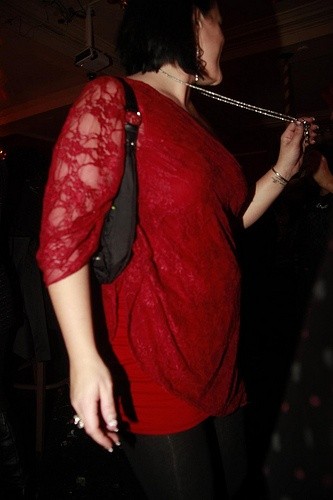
[159,68,311,143]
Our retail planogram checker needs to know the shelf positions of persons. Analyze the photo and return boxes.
[37,0,318,500]
[0,174,51,500]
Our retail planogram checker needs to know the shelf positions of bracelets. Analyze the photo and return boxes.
[271,167,289,183]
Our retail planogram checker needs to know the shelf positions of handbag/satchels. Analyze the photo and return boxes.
[92,77,143,285]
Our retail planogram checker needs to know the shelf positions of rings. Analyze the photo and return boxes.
[72,415,84,429]
